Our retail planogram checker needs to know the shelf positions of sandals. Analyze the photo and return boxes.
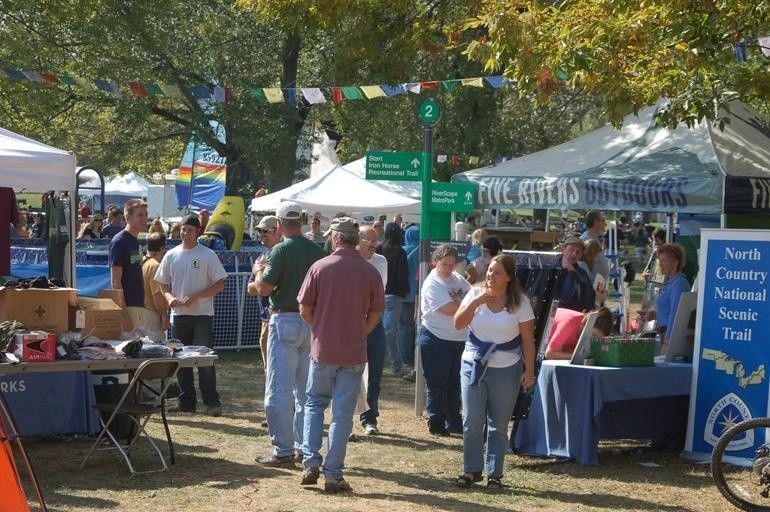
[488,476,503,488]
[458,472,484,487]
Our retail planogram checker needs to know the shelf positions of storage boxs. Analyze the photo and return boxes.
[74,290,122,339]
[1,287,80,337]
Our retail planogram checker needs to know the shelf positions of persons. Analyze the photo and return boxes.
[453,256,535,491]
[545,306,614,365]
[463,228,503,286]
[579,239,602,285]
[252,202,329,469]
[466,208,581,242]
[307,210,406,252]
[556,237,596,313]
[417,245,474,438]
[247,215,280,427]
[11,203,45,239]
[604,213,667,266]
[642,244,691,355]
[141,233,180,397]
[109,199,148,341]
[578,209,610,289]
[399,225,420,373]
[375,225,418,380]
[349,226,387,437]
[78,204,211,239]
[154,215,228,417]
[298,216,386,492]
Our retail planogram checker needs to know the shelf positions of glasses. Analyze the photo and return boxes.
[257,229,277,234]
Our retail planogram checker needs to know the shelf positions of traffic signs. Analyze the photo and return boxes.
[366,150,423,181]
[431,182,477,212]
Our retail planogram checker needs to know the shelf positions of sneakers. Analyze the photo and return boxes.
[428,423,449,436]
[348,433,359,443]
[363,422,379,434]
[403,370,417,382]
[255,454,295,468]
[206,401,223,417]
[300,466,319,485]
[168,401,197,415]
[325,478,353,493]
[261,420,270,427]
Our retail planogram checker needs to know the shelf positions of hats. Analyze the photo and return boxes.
[253,215,282,230]
[322,217,360,238]
[276,200,301,220]
[179,214,200,226]
[564,236,583,246]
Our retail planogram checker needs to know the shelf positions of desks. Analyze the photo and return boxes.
[1,344,215,467]
[512,360,696,469]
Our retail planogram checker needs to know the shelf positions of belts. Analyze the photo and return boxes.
[270,307,293,314]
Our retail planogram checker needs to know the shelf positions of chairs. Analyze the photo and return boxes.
[0,395,46,512]
[78,358,187,476]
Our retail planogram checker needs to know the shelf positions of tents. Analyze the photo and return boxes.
[0,123,77,193]
[91,170,153,214]
[251,147,438,223]
[450,92,769,229]
